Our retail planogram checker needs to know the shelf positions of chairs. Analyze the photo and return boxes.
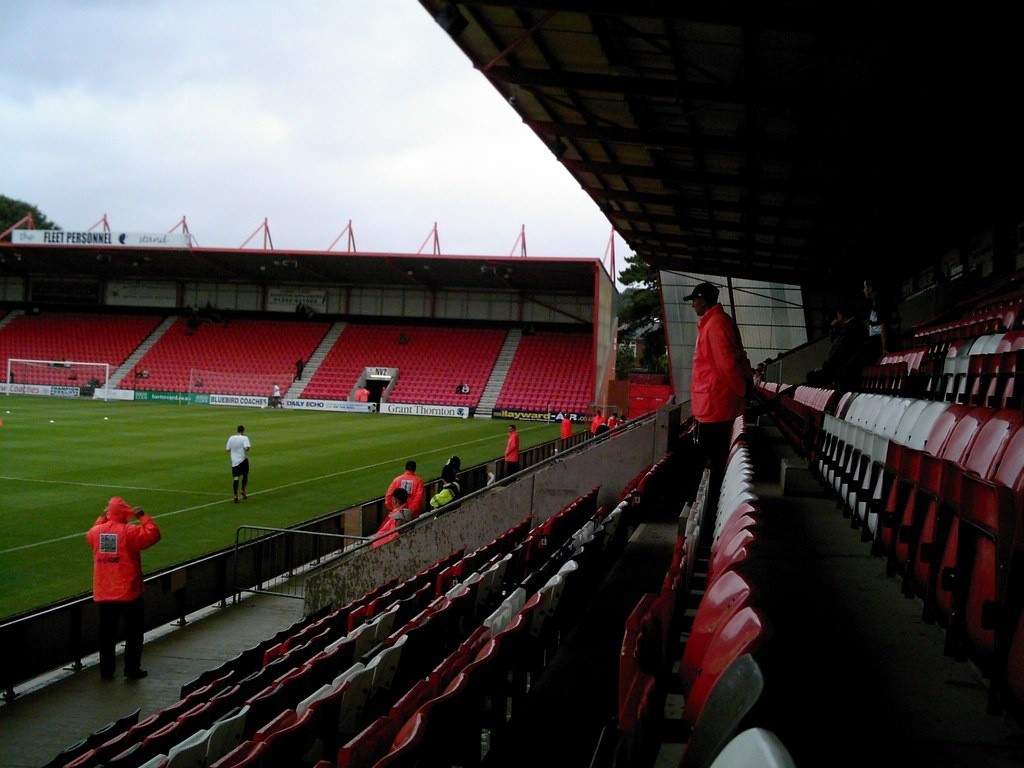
[0,268,1024,768]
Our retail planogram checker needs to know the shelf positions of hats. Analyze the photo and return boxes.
[449,456,460,472]
[389,508,412,521]
[405,461,416,469]
[683,283,720,302]
[441,465,455,483]
[389,488,409,501]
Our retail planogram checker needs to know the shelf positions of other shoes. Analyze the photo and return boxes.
[124,670,147,679]
[103,674,114,680]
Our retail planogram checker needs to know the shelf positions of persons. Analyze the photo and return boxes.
[681,283,754,489]
[136,367,149,379]
[561,414,572,452]
[225,425,250,503]
[68,370,78,380]
[85,496,161,680]
[88,375,100,388]
[751,358,773,386]
[455,384,470,394]
[354,385,386,402]
[273,381,282,408]
[803,282,901,394]
[591,410,628,438]
[505,424,519,476]
[296,358,303,380]
[373,456,462,549]
[194,374,203,387]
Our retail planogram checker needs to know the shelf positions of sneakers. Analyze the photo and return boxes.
[234,496,238,503]
[241,490,248,500]
